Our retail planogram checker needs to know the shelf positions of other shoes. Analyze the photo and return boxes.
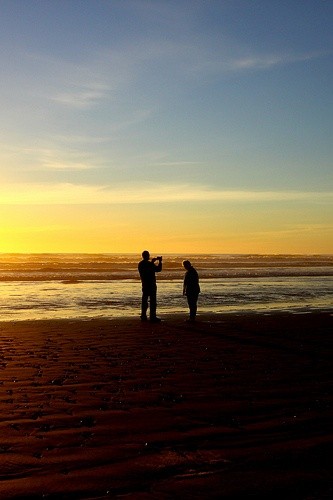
[150,317,161,322]
[138,316,146,321]
[184,317,196,323]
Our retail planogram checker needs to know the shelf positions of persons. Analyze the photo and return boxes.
[182,260,200,323]
[137,250,163,322]
